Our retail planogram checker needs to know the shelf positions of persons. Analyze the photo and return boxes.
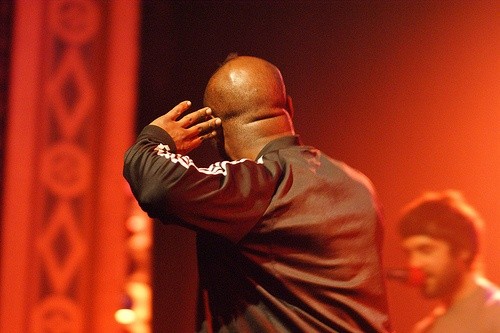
[398,190,500,333]
[123,57,389,332]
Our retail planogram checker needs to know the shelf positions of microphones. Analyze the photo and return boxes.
[385,269,424,287]
